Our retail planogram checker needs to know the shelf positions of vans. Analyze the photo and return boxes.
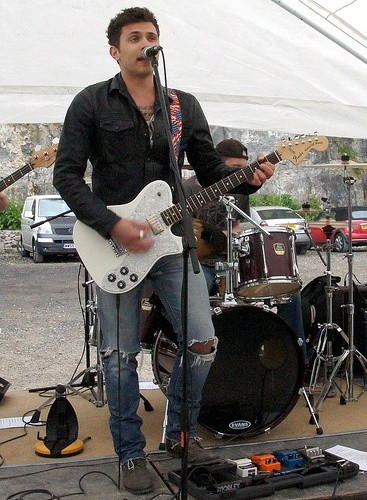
[20,194,80,263]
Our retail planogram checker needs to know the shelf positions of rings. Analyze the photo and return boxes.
[139,230,143,240]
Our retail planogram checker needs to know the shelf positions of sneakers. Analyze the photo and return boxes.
[121,457,155,495]
[166,435,221,465]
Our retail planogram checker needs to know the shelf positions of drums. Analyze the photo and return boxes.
[89,217,232,350]
[151,300,305,439]
[229,226,304,301]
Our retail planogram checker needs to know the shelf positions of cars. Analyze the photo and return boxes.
[239,205,312,254]
[308,206,367,252]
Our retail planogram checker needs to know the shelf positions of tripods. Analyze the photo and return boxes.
[305,167,367,424]
[29,209,154,423]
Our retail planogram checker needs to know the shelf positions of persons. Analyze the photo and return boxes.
[183,138,251,290]
[53,8,276,493]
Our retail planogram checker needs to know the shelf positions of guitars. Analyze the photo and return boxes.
[0,144,59,192]
[73,131,329,294]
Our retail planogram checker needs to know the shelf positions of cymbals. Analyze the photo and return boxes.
[300,160,367,168]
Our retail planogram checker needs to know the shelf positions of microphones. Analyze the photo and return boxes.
[142,46,162,61]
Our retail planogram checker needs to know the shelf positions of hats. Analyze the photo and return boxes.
[215,138,249,161]
